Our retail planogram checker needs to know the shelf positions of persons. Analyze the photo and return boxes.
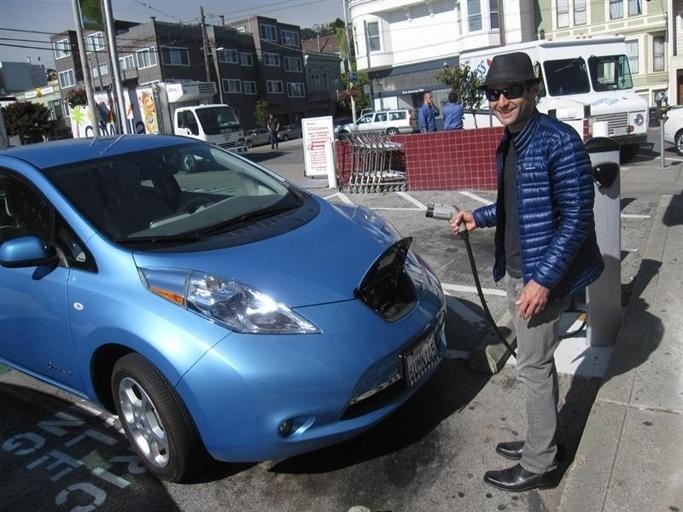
[442,93,464,129]
[418,90,440,133]
[267,111,280,149]
[448,52,605,492]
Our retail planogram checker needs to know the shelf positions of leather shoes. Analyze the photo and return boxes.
[483,464,558,493]
[495,441,524,461]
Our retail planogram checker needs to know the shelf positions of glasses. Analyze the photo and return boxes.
[485,84,523,101]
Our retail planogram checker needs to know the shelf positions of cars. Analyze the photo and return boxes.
[0,133,450,484]
[277,123,302,141]
[244,128,271,148]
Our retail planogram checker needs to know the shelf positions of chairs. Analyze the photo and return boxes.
[0,160,171,263]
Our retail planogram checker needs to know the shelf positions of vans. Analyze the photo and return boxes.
[334,109,418,143]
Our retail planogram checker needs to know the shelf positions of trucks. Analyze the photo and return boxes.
[66,78,250,169]
[459,33,650,161]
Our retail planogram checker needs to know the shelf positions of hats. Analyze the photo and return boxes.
[475,51,542,89]
